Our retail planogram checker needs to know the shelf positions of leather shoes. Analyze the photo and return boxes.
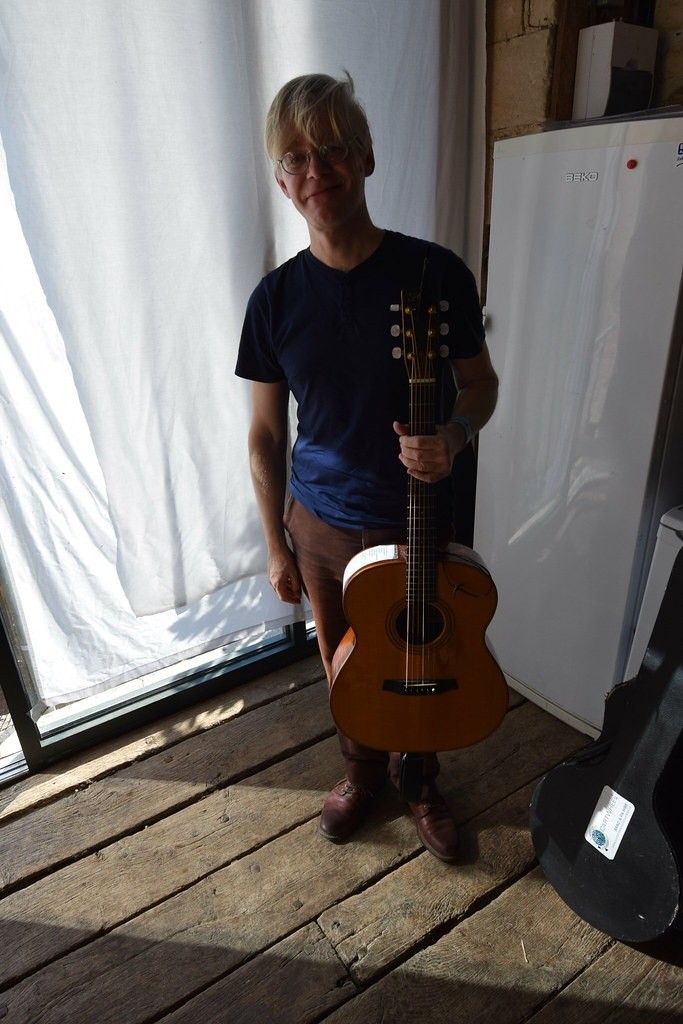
[407,782,462,862]
[320,777,374,841]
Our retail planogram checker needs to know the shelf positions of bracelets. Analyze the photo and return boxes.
[449,417,471,443]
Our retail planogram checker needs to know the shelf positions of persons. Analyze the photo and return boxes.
[235,73,499,863]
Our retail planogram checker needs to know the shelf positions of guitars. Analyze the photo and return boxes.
[329,286,512,753]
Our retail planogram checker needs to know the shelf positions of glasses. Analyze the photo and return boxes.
[278,141,354,176]
